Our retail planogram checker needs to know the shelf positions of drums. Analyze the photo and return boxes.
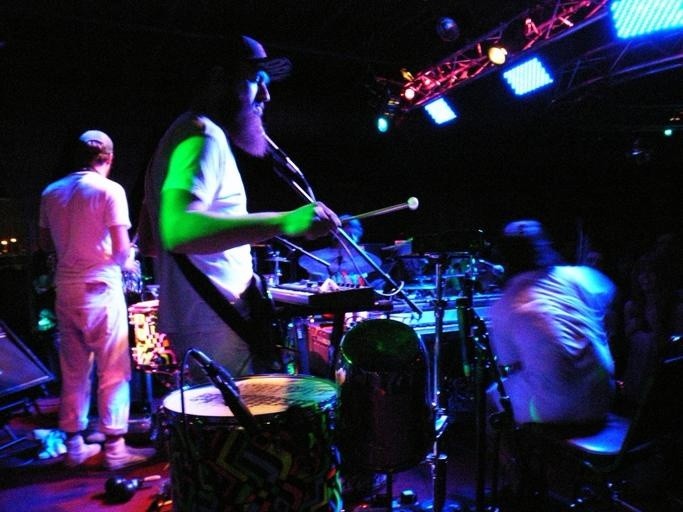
[129,300,179,375]
[336,319,433,474]
[161,373,344,512]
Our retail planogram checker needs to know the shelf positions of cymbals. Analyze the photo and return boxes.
[300,248,381,277]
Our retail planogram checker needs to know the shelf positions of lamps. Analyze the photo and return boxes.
[372,97,411,135]
[487,30,523,66]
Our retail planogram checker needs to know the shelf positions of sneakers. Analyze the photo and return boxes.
[64,435,102,465]
[103,438,157,471]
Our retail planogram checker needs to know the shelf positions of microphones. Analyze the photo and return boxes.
[266,136,303,177]
[190,346,241,401]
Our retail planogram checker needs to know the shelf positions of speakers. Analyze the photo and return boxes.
[0,321,53,405]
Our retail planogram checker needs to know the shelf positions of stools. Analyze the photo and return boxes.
[564,410,639,512]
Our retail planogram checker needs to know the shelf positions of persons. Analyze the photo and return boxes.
[34,129,158,472]
[148,33,343,382]
[490,218,626,511]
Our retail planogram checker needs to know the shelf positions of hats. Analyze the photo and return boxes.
[201,36,294,80]
[79,130,114,155]
